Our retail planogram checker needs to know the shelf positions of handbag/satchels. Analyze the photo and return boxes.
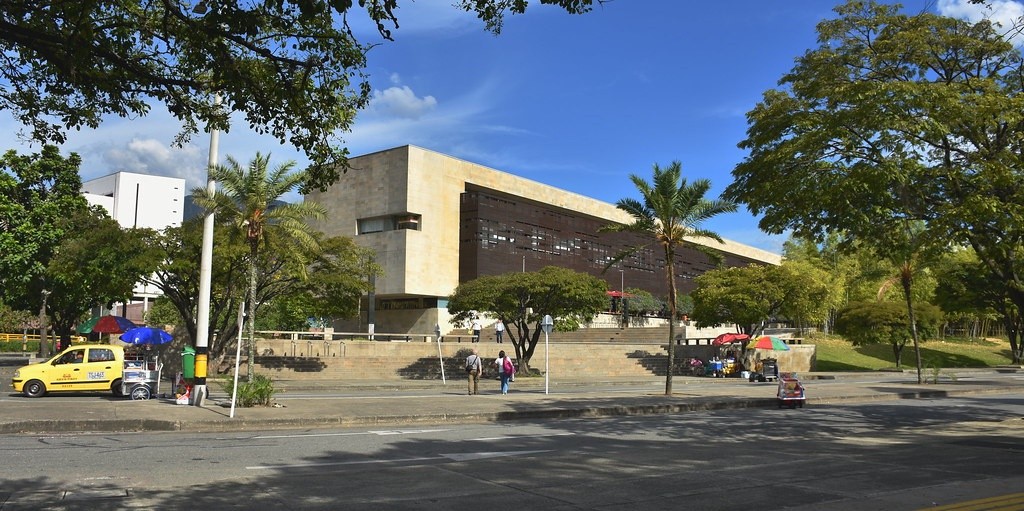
[465,365,473,372]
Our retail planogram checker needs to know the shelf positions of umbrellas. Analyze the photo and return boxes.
[744,335,790,357]
[119,327,173,354]
[606,290,636,298]
[77,314,137,334]
[712,333,750,347]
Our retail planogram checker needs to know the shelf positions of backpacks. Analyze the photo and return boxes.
[503,356,516,377]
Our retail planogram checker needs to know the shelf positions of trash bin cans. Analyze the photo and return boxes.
[180,346,195,379]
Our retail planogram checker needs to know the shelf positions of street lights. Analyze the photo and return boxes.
[618,269,624,327]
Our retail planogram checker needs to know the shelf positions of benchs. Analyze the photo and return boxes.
[255,330,479,343]
[674,337,805,345]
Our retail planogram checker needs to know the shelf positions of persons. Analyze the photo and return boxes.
[682,314,691,326]
[467,349,482,395]
[434,324,441,342]
[495,319,505,343]
[495,350,514,396]
[469,316,482,343]
[67,352,84,364]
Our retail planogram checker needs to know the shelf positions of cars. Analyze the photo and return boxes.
[9,344,127,398]
